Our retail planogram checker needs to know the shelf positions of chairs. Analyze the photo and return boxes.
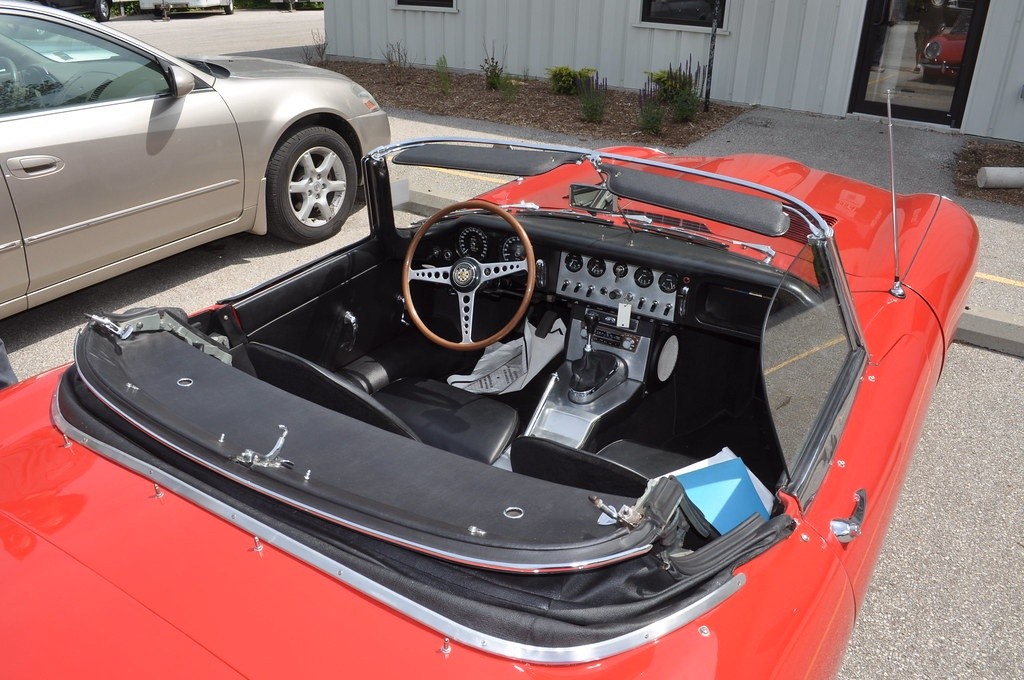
[245,340,518,465]
[509,434,700,496]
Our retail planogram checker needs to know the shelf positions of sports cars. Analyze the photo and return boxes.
[0,137,979,680]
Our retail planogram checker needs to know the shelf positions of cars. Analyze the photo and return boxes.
[139,0,234,17]
[0,0,391,321]
[7,0,112,39]
